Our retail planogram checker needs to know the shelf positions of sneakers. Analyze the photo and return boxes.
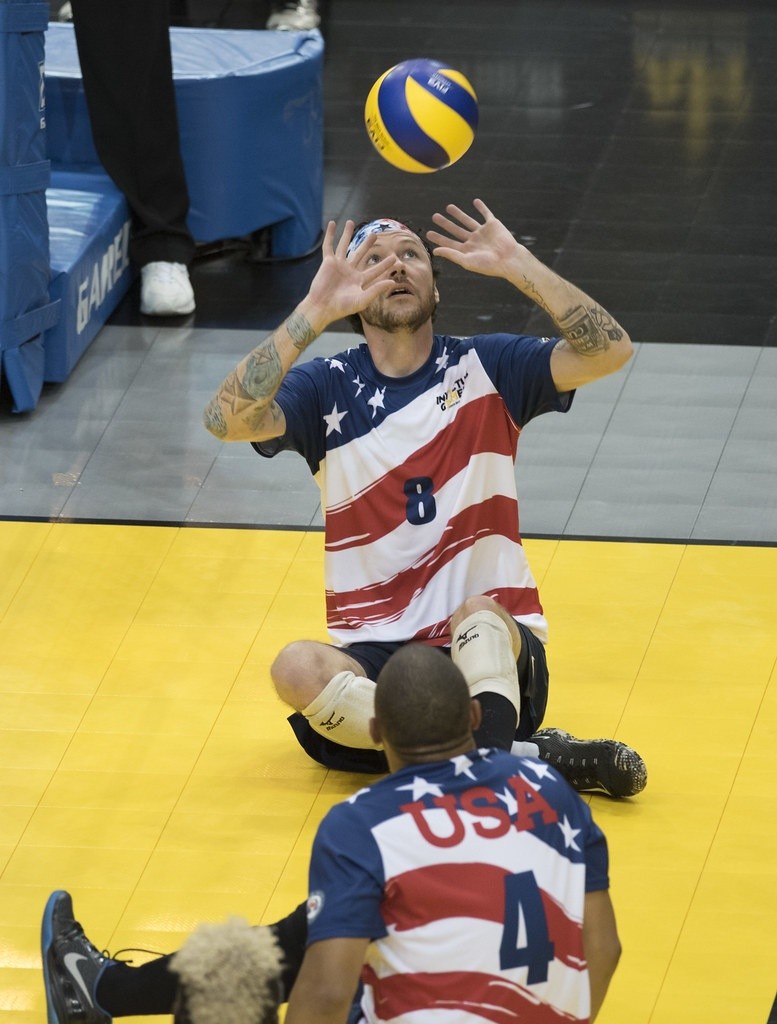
[527,728,647,798]
[41,891,169,1024]
[139,260,196,317]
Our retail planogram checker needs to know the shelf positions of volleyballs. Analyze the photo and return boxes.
[365,60,482,175]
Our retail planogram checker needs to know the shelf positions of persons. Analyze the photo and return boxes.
[70,0,196,318]
[41,639,622,1024]
[202,197,648,798]
